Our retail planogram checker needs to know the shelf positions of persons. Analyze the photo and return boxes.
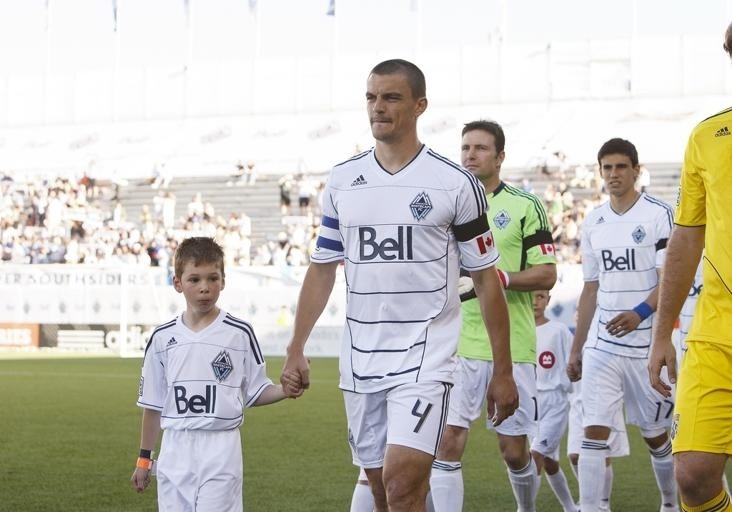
[527,285,576,511]
[428,120,558,511]
[565,373,633,511]
[286,60,519,511]
[345,466,437,512]
[131,236,303,511]
[647,26,731,512]
[567,138,682,511]
[658,242,732,502]
[0,161,253,269]
[254,172,328,269]
[520,145,650,267]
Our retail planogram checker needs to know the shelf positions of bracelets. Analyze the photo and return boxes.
[632,301,651,320]
[137,457,154,469]
[498,270,511,288]
[138,448,155,458]
